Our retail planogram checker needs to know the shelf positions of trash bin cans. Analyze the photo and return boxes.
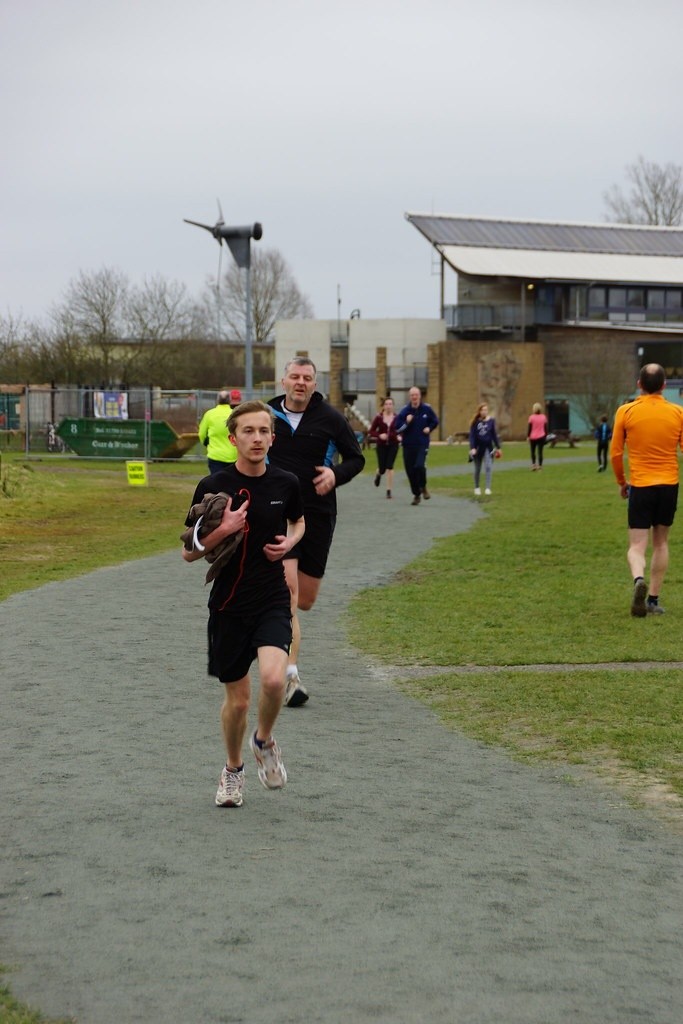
[545,399,570,433]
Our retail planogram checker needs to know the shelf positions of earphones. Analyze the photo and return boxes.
[232,438,234,443]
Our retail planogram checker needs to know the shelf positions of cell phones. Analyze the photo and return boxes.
[229,492,246,512]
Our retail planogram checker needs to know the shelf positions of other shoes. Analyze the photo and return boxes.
[474,487,481,495]
[631,578,648,618]
[420,488,430,499]
[646,603,665,615]
[485,488,492,495]
[374,474,380,487]
[411,496,420,505]
[598,465,606,472]
[386,489,392,499]
[531,463,543,471]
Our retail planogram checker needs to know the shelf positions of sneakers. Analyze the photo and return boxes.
[284,672,309,707]
[215,765,246,807]
[249,726,287,790]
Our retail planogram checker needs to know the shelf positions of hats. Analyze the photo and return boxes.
[230,389,241,405]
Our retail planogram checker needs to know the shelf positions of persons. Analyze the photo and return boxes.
[368,399,400,498]
[230,390,241,409]
[393,386,439,505]
[595,417,612,472]
[180,401,305,808]
[198,391,237,476]
[526,399,576,471]
[610,364,683,618]
[0,409,6,430]
[469,403,502,495]
[262,357,365,707]
